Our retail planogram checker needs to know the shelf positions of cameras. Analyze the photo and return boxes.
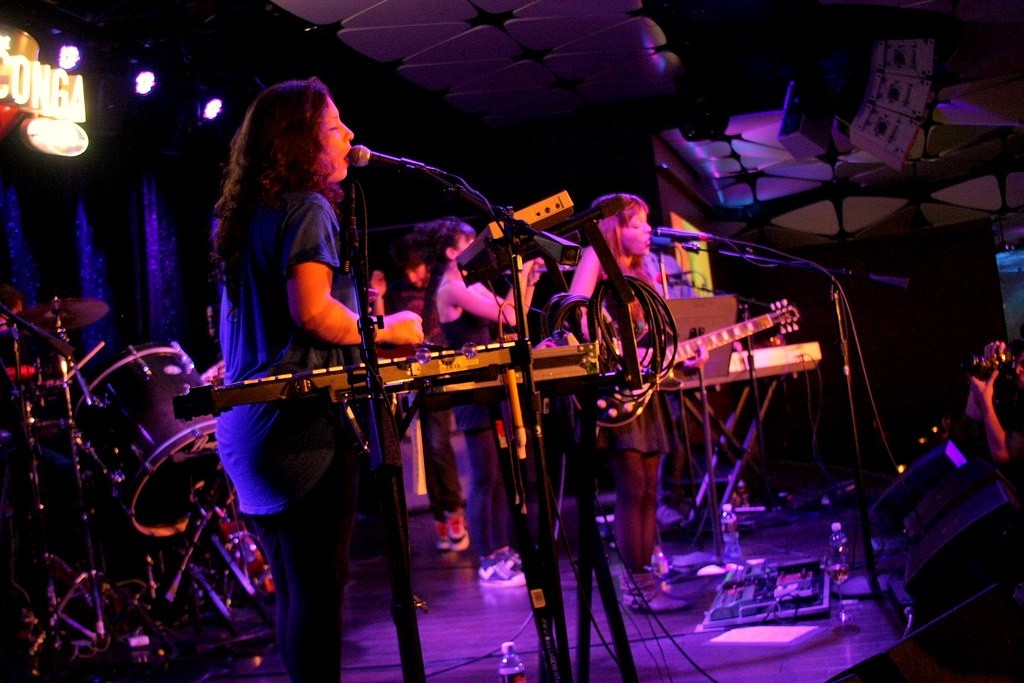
[961,353,1016,391]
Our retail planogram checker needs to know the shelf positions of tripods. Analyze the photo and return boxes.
[9,314,181,670]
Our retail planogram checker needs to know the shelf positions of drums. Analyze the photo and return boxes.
[25,332,74,388]
[70,337,220,539]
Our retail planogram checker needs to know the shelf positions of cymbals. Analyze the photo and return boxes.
[21,296,110,334]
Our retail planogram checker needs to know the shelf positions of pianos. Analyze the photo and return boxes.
[674,339,824,550]
[170,337,518,423]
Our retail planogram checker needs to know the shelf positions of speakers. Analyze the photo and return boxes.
[847,36,935,170]
[869,437,1024,676]
[777,79,837,161]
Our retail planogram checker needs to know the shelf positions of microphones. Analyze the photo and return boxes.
[348,146,443,178]
[653,227,721,244]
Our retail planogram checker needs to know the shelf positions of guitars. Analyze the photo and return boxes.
[568,298,802,428]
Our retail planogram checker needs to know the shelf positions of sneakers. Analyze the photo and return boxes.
[446,510,470,551]
[432,518,453,551]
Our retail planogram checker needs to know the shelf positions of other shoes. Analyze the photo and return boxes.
[479,548,527,587]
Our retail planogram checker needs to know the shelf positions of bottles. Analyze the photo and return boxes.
[651,546,672,593]
[829,521,850,582]
[720,504,740,558]
[497,642,526,683]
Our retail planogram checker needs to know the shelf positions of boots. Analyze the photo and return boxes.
[622,564,688,613]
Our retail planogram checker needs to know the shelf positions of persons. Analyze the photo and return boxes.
[363,191,708,611]
[215,78,423,683]
[874,338,1024,496]
